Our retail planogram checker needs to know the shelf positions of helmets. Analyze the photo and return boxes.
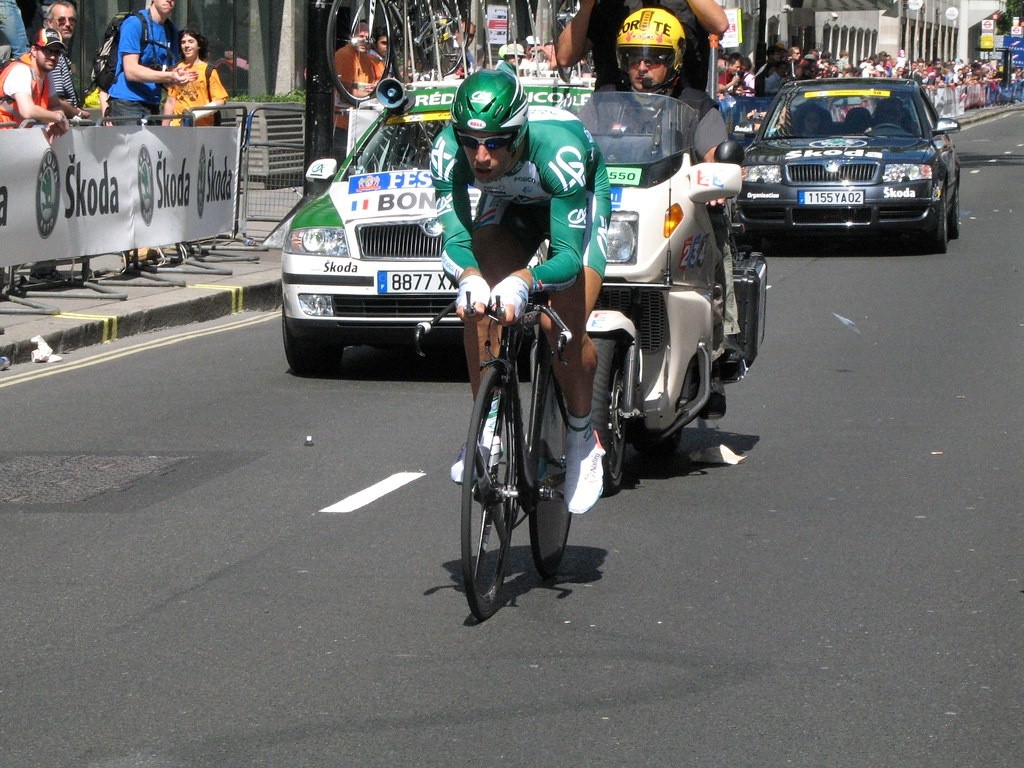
[451,71,528,153]
[616,7,685,74]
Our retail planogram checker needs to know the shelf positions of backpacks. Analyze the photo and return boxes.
[91,11,175,91]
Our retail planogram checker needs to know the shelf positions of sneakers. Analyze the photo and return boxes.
[0,272,26,286]
[123,247,158,261]
[29,268,69,283]
[562,428,606,514]
[450,434,505,485]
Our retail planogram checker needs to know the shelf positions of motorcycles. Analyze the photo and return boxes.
[569,91,768,497]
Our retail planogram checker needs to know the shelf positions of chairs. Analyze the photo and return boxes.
[844,107,872,133]
[796,107,833,135]
[872,108,915,133]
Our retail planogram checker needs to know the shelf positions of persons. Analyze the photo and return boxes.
[557,0,730,93]
[331,16,594,176]
[575,8,744,368]
[429,71,612,514]
[716,41,1024,120]
[0,0,229,296]
[791,106,823,136]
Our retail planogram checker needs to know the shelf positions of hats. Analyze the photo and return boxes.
[526,35,541,45]
[503,44,526,58]
[804,54,818,62]
[31,27,66,48]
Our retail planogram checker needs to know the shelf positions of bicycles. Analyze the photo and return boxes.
[312,0,469,105]
[413,288,575,621]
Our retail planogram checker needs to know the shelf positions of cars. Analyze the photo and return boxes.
[281,75,594,380]
[732,76,962,255]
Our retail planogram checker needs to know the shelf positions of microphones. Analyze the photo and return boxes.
[642,70,680,89]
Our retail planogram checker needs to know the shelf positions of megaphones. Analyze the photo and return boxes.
[375,77,405,108]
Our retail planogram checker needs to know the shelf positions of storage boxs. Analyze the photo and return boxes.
[732,250,767,365]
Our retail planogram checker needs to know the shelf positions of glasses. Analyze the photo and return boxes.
[623,51,673,69]
[43,45,64,57]
[48,17,78,26]
[804,116,822,123]
[880,108,903,116]
[530,44,540,47]
[454,128,517,150]
[806,59,815,63]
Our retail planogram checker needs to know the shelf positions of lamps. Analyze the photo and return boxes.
[781,4,794,13]
